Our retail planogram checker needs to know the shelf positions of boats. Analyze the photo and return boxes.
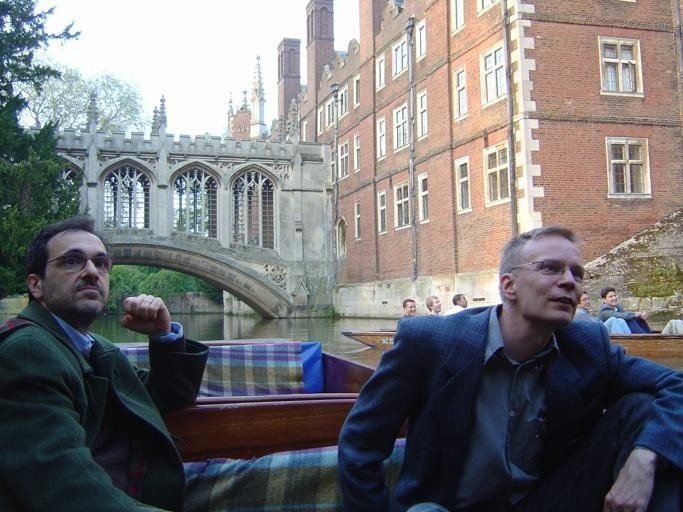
[340,331,682,372]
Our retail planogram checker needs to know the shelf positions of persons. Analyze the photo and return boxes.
[597,287,651,334]
[336,226,682,512]
[424,296,441,316]
[443,294,467,317]
[0,212,209,512]
[395,297,416,331]
[572,291,631,334]
[659,319,682,334]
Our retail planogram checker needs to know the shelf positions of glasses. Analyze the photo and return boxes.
[46,254,113,273]
[505,260,591,283]
